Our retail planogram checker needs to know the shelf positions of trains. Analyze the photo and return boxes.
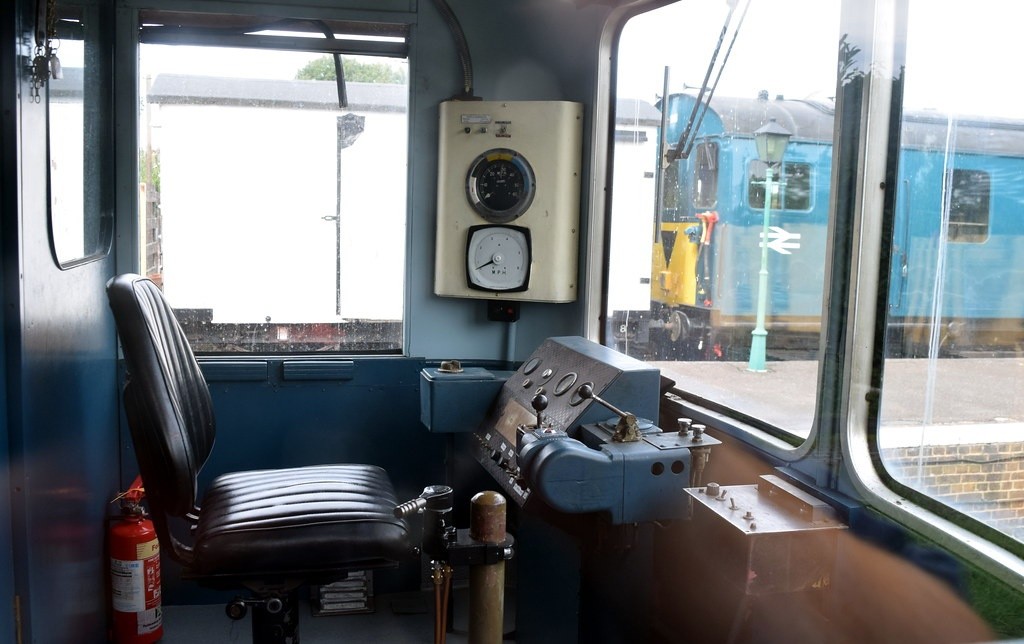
[652,93,1024,348]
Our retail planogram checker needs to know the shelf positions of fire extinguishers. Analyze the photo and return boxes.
[106,472,162,644]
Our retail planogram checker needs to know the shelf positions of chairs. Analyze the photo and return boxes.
[105,273,413,644]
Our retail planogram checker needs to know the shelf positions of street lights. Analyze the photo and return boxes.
[747,116,793,372]
